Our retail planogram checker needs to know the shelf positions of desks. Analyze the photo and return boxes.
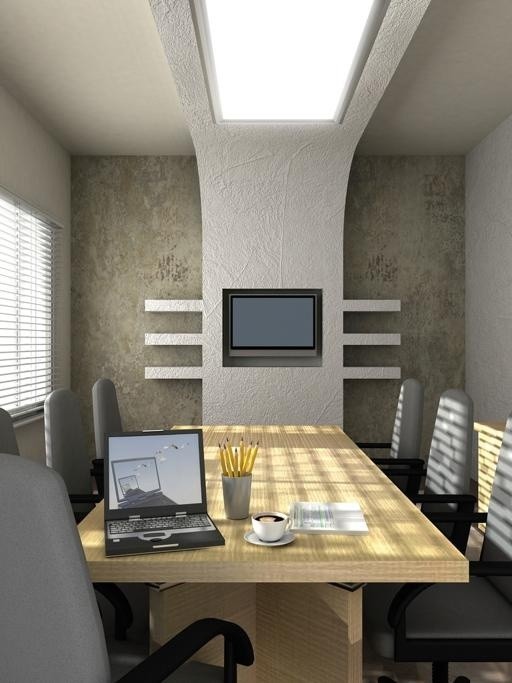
[69,423,470,682]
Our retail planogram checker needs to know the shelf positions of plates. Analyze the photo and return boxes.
[244,531,294,547]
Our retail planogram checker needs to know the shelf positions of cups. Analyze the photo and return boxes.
[222,469,251,520]
[250,510,292,539]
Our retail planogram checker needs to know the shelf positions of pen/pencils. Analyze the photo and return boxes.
[218,437,259,477]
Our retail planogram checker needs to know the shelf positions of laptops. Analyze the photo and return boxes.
[119,475,145,496]
[104,429,225,558]
[110,457,177,508]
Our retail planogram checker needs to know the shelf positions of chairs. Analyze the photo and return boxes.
[85,376,125,488]
[0,451,256,682]
[357,378,424,493]
[381,386,479,556]
[0,406,137,656]
[380,416,512,682]
[36,387,106,511]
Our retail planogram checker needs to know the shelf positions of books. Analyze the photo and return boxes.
[283,499,370,537]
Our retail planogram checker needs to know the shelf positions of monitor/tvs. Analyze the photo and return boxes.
[222,288,322,367]
